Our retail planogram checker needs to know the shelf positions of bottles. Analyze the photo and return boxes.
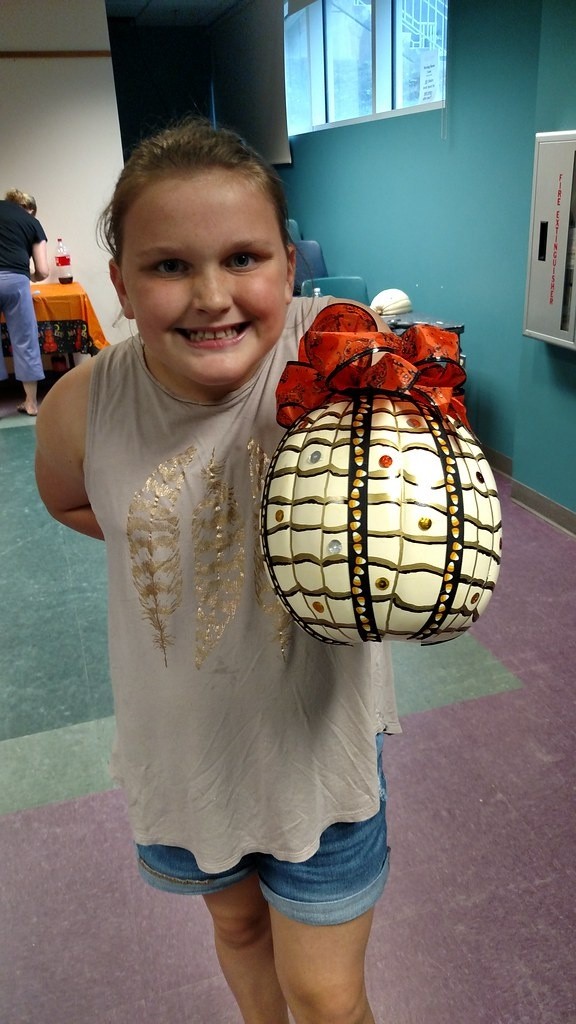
[55,239,74,284]
[311,288,323,298]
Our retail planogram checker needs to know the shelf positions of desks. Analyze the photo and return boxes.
[0,281,112,375]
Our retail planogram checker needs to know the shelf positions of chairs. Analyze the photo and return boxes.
[284,218,370,306]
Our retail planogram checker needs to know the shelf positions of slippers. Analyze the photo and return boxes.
[17,404,39,416]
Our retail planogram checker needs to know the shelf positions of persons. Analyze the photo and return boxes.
[35,122,396,1024]
[0,189,49,417]
